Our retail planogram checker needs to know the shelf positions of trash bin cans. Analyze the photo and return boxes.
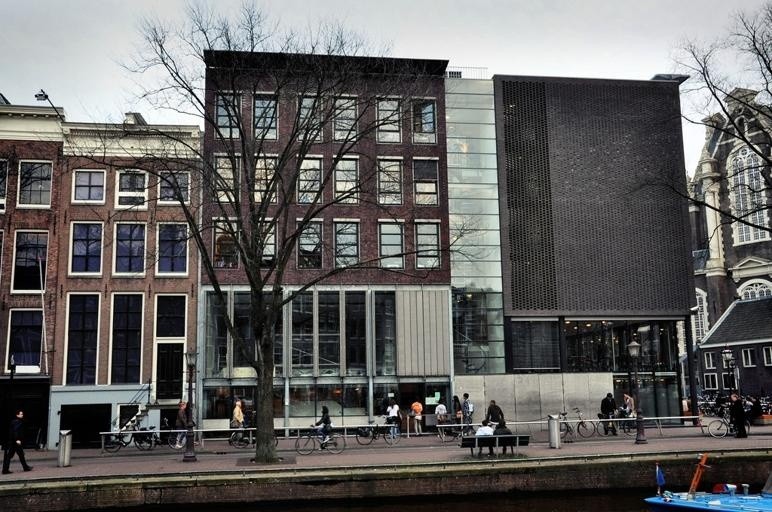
[548,415,561,449]
[57,430,72,467]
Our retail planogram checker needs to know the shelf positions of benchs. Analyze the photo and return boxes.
[458,435,532,459]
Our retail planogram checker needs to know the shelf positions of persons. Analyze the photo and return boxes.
[601,392,636,436]
[461,393,471,433]
[743,396,772,423]
[485,398,505,424]
[729,393,747,438]
[386,399,402,434]
[681,395,692,412]
[174,401,200,449]
[474,419,494,455]
[2,408,34,474]
[492,419,513,454]
[312,405,332,451]
[453,395,462,430]
[228,399,244,446]
[434,398,447,439]
[411,395,423,435]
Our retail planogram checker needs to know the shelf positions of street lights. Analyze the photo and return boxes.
[627,337,648,444]
[182,346,199,462]
[722,348,733,400]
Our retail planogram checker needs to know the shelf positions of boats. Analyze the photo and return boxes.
[644,455,772,512]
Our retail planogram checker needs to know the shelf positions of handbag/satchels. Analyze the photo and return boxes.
[231,419,238,429]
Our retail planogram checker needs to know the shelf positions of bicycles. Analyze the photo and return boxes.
[231,411,278,449]
[140,418,187,449]
[597,407,638,437]
[438,411,482,442]
[104,420,152,452]
[695,393,772,419]
[706,409,751,438]
[559,407,595,437]
[356,414,402,445]
[295,424,346,455]
